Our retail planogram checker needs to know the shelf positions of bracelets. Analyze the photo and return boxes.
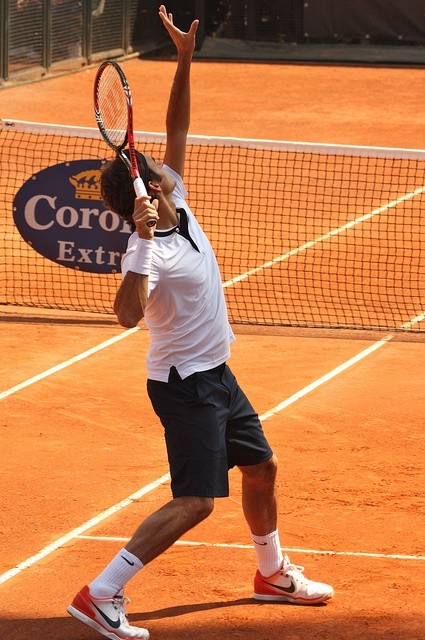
[128,238,155,277]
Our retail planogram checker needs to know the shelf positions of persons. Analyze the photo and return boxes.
[67,4,335,640]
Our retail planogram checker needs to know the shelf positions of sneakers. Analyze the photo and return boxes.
[254,555,334,605]
[66,585,150,640]
